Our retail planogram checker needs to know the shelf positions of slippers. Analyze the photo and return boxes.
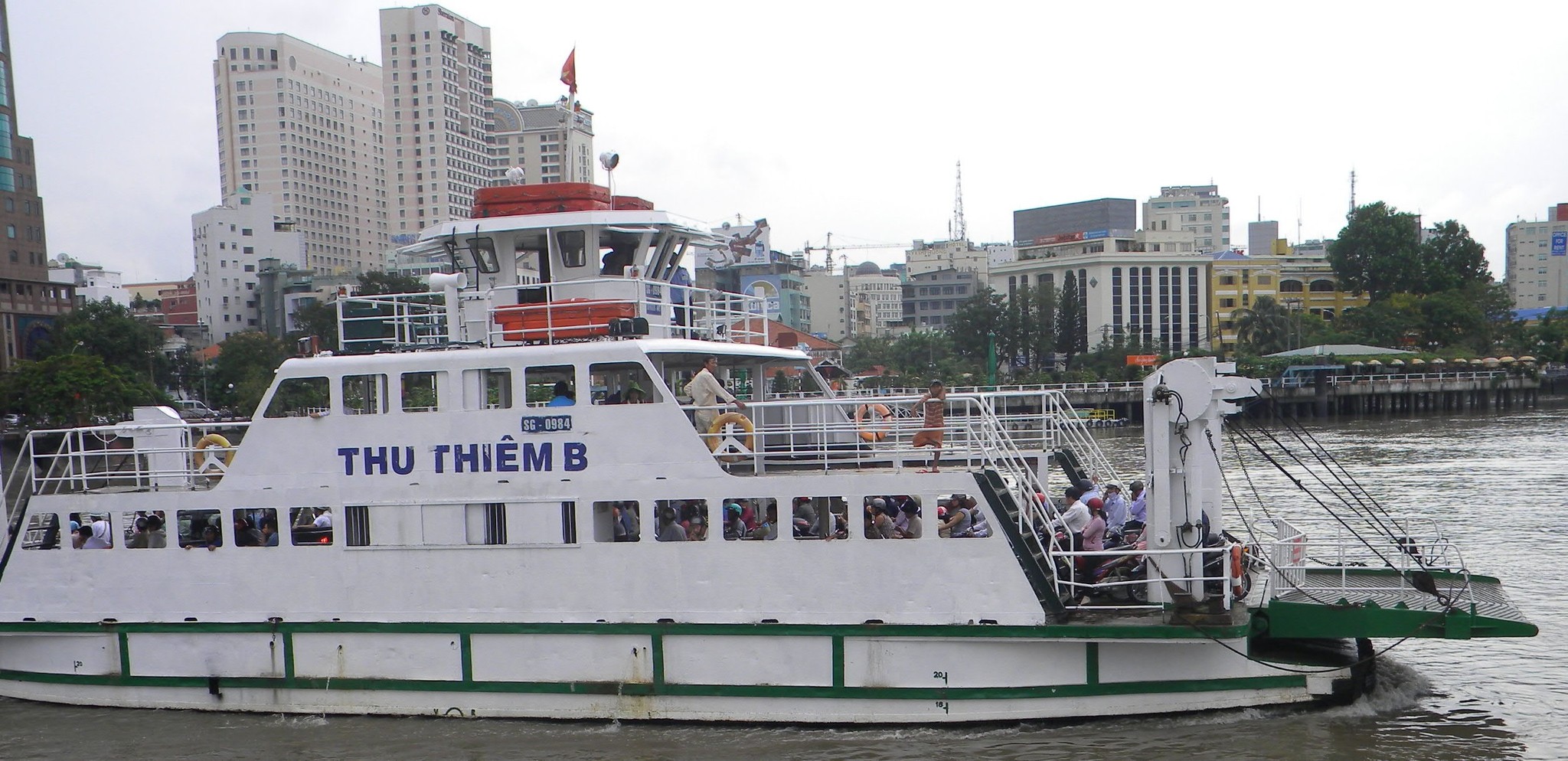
[932,469,940,473]
[915,469,927,473]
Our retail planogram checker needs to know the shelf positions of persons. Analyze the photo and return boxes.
[938,494,988,538]
[613,501,639,542]
[310,394,355,417]
[128,511,165,548]
[656,500,709,541]
[621,382,646,404]
[723,497,777,540]
[1004,477,1210,597]
[864,495,922,539]
[545,381,575,406]
[683,355,747,475]
[793,496,848,542]
[663,252,699,339]
[221,406,241,418]
[179,517,223,550]
[296,506,332,529]
[911,379,945,472]
[70,512,111,549]
[234,514,278,546]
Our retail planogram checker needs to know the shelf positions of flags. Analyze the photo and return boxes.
[561,49,577,94]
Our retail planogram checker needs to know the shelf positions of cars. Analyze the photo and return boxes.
[3,414,19,429]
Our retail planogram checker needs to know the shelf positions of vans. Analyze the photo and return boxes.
[174,400,215,419]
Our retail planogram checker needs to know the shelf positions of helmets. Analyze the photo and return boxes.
[689,515,705,526]
[660,507,676,519]
[203,524,220,536]
[1032,483,1041,493]
[146,514,161,526]
[870,497,886,511]
[1129,480,1144,491]
[725,503,742,517]
[234,518,249,527]
[1085,496,1103,510]
[1032,493,1046,504]
[684,505,700,516]
[135,517,148,528]
[1075,478,1093,491]
[1105,480,1122,492]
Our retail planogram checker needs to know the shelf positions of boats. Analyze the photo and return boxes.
[0,149,1542,734]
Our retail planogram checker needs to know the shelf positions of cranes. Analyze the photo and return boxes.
[802,230,915,273]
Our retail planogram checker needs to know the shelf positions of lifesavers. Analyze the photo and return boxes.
[707,412,753,463]
[1087,420,1093,427]
[1105,420,1111,427]
[1025,424,1032,429]
[1013,423,1018,429]
[955,429,964,432]
[998,424,1005,429]
[1068,421,1075,429]
[1060,423,1066,430]
[1113,422,1118,427]
[1096,420,1103,427]
[1119,420,1126,427]
[194,434,233,480]
[1047,420,1050,428]
[1232,544,1243,596]
[854,404,892,441]
[1077,421,1084,428]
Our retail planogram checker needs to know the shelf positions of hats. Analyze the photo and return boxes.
[900,500,918,513]
[797,498,809,500]
[950,494,966,500]
[622,382,647,399]
[937,506,949,516]
[612,506,620,518]
[737,498,749,502]
[311,507,326,511]
[907,495,921,507]
[88,511,106,517]
[929,379,943,390]
[69,520,80,530]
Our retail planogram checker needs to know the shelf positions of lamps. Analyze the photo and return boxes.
[505,166,526,186]
[717,324,727,338]
[599,150,619,197]
[629,264,639,279]
[711,288,723,300]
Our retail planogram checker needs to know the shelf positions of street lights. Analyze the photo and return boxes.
[228,383,242,433]
[195,316,211,420]
